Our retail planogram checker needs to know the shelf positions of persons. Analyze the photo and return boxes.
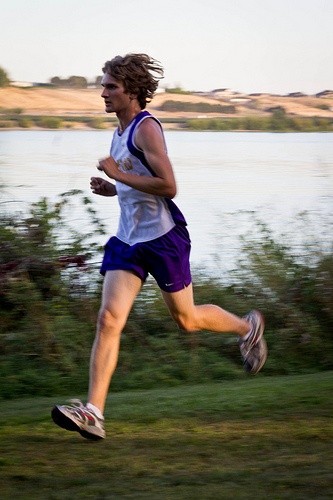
[51,54,268,442]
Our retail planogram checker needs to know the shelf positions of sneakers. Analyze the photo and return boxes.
[50,404,106,440]
[240,309,267,375]
